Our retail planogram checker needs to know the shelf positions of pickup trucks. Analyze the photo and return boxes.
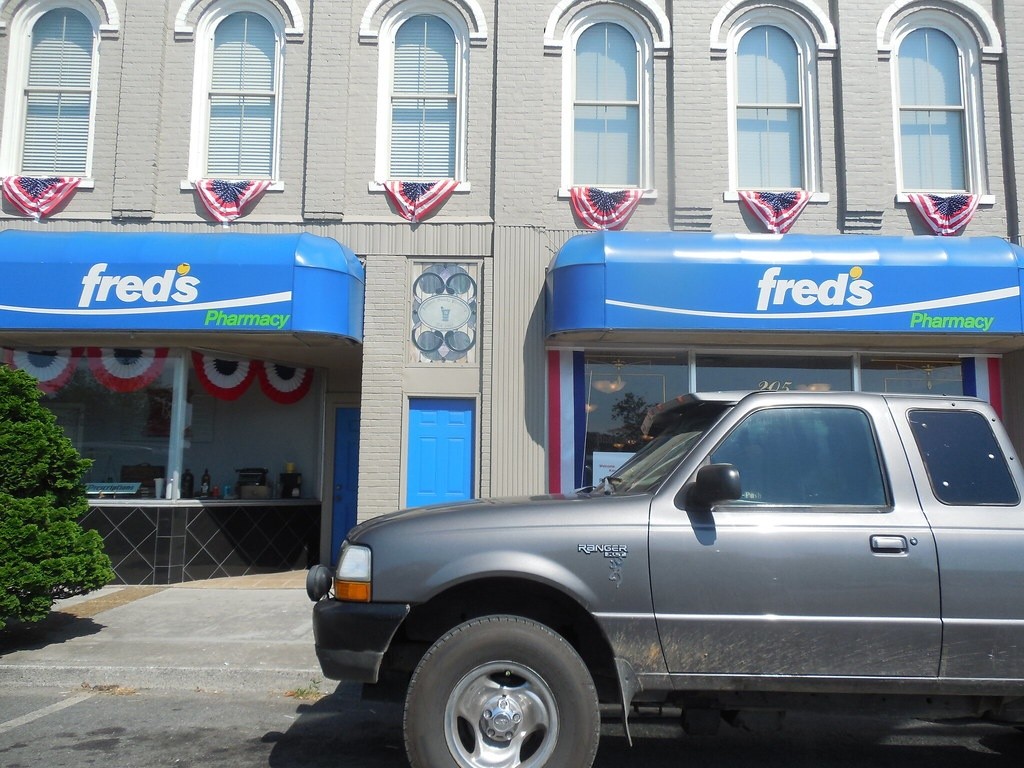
[306,390,1024,768]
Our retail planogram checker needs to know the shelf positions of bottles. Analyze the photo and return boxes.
[287,462,296,473]
[154,478,164,499]
[225,485,232,496]
[201,469,210,495]
[212,485,218,496]
[181,469,194,498]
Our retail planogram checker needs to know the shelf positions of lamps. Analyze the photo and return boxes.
[584,403,597,414]
[592,380,625,393]
